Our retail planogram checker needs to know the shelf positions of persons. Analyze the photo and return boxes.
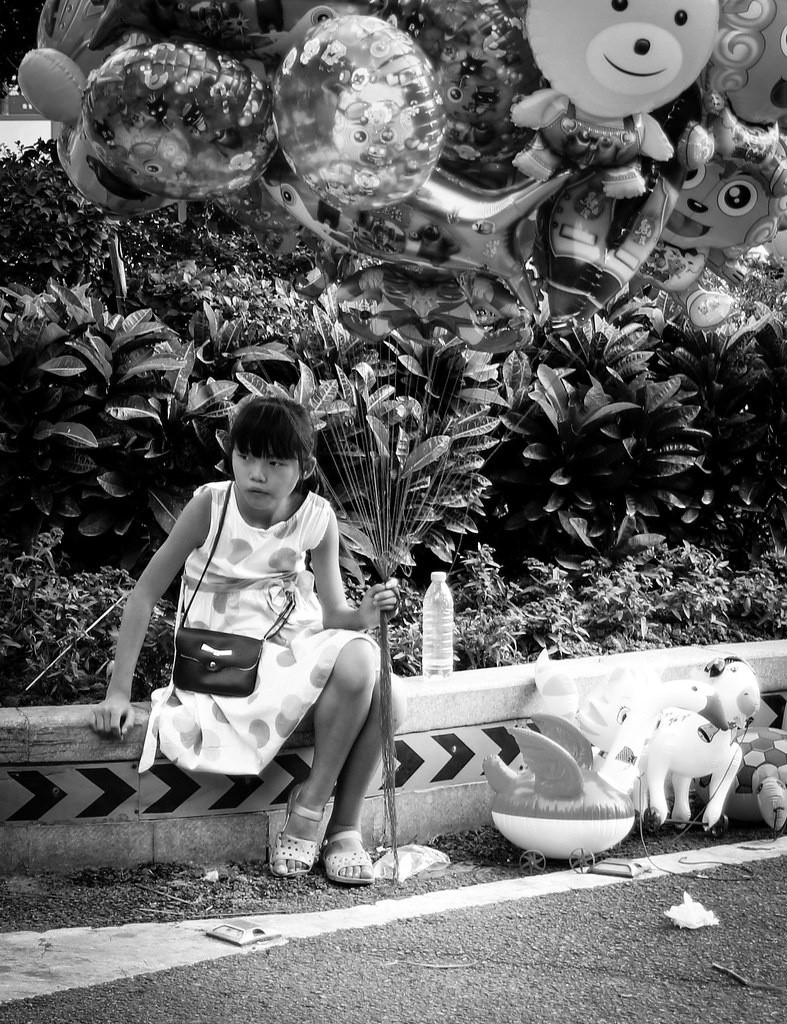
[86,398,407,885]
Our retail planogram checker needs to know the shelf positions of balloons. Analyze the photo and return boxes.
[17,0,787,356]
[483,646,787,874]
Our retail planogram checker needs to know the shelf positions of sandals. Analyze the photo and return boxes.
[268,781,325,878]
[319,831,374,884]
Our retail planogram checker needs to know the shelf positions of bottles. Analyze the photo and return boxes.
[422,572,454,683]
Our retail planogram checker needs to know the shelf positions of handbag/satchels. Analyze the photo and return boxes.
[173,626,263,698]
